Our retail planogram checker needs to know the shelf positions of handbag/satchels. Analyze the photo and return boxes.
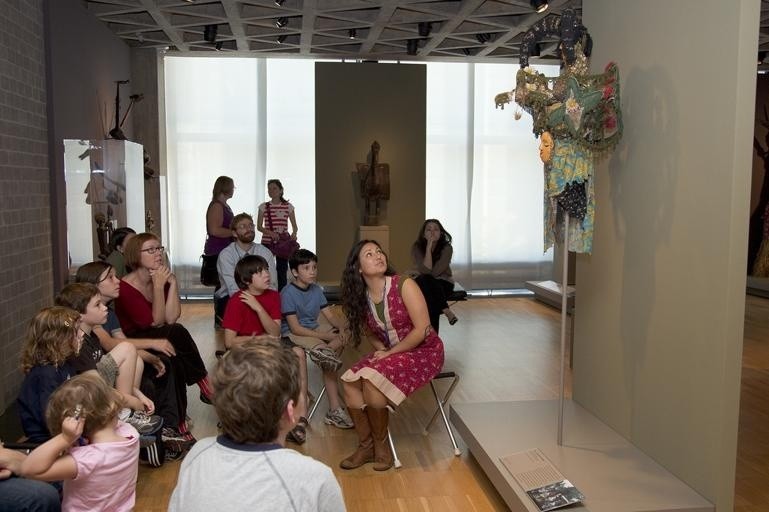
[198,254,222,287]
[272,232,301,259]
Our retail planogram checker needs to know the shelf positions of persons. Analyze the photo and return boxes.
[165,330,348,512]
[278,248,355,429]
[212,211,278,321]
[199,176,236,329]
[1,225,217,511]
[339,239,445,471]
[257,179,298,294]
[539,131,555,164]
[407,218,458,337]
[221,255,309,445]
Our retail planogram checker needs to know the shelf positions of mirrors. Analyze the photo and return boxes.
[63,139,145,276]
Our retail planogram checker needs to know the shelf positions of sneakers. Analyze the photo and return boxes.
[323,408,355,431]
[122,413,164,436]
[309,345,343,374]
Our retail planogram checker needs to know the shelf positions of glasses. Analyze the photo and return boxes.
[239,223,256,229]
[140,246,165,254]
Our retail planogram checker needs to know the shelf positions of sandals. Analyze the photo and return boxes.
[288,416,310,446]
[446,309,459,326]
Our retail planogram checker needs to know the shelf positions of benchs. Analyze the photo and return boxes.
[321,282,468,307]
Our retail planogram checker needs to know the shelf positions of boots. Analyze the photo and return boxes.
[364,406,396,472]
[339,404,375,469]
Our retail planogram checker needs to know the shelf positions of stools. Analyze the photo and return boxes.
[386,372,461,467]
